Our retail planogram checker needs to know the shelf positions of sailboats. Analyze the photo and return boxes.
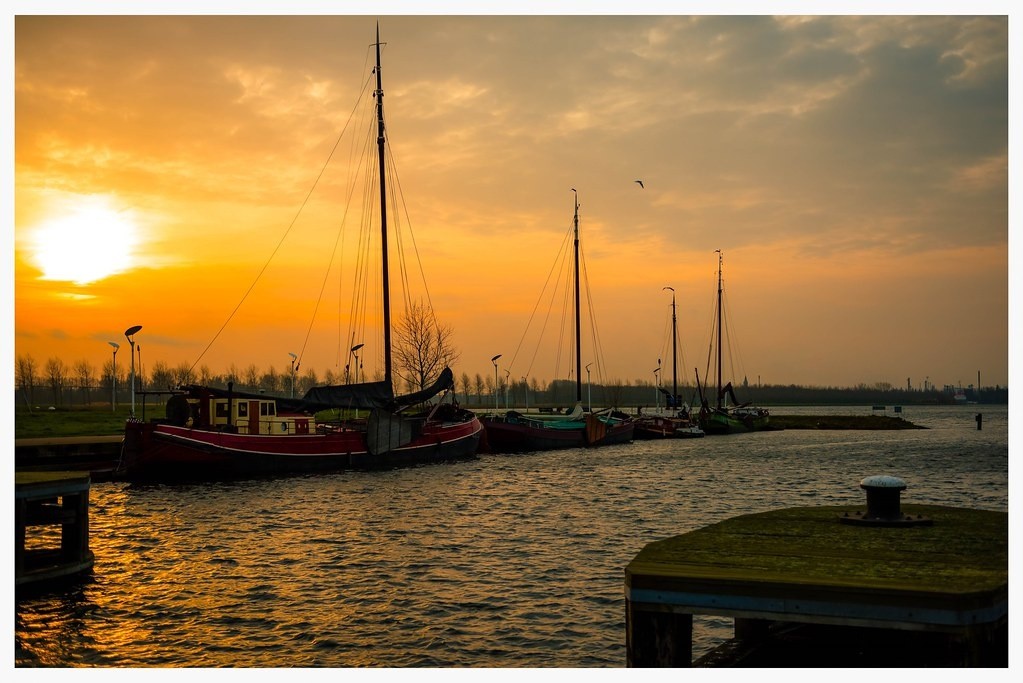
[113,22,490,477]
[470,186,633,450]
[696,250,772,431]
[628,287,705,438]
